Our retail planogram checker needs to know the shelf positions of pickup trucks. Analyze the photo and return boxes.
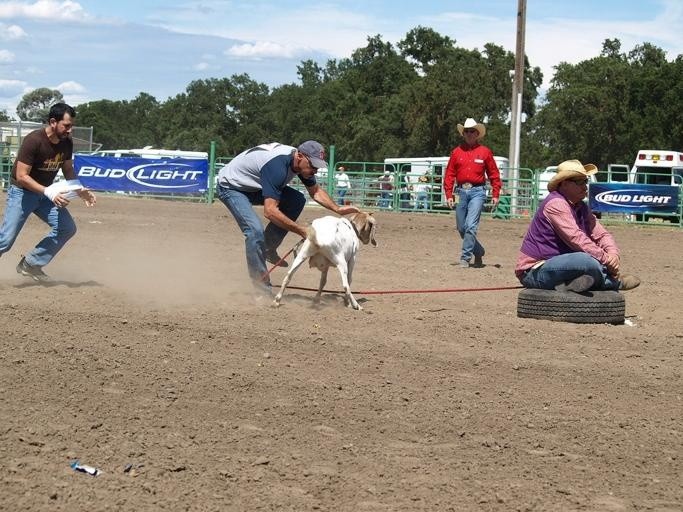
[531,166,601,218]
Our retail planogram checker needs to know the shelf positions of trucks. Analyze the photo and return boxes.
[380,156,507,208]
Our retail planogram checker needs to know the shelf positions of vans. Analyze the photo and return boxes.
[607,150,683,224]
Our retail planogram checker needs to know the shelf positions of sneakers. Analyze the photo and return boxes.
[618,274,640,291]
[555,274,593,293]
[459,259,469,268]
[15,254,50,283]
[264,250,288,267]
[251,294,279,309]
[475,249,485,268]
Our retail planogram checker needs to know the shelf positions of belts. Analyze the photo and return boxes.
[456,182,485,189]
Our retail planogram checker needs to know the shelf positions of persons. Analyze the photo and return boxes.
[443,116,502,270]
[516,159,641,294]
[1,102,96,287]
[334,164,442,213]
[216,139,360,304]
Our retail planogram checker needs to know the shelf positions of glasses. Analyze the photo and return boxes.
[566,177,589,186]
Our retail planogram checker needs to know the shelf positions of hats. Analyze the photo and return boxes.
[299,140,327,168]
[547,159,598,191]
[456,118,485,139]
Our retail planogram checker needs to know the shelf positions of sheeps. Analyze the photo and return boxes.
[269,210,378,312]
